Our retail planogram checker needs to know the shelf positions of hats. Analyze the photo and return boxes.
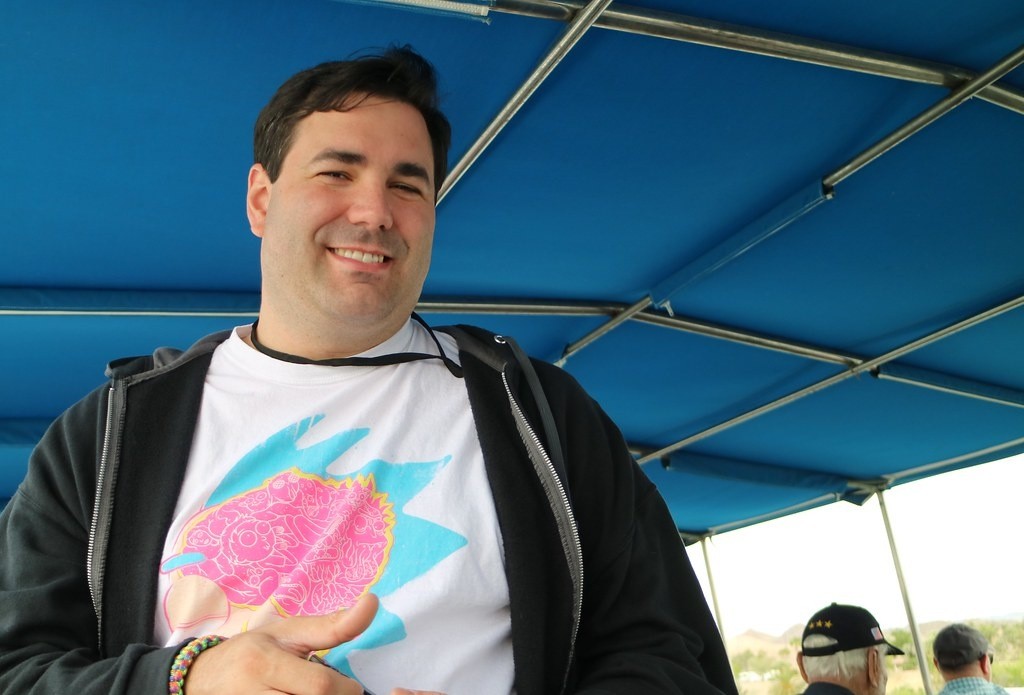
[801,602,905,656]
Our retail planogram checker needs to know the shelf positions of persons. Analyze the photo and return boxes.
[0,42,739,695]
[790,603,906,694]
[932,624,1008,694]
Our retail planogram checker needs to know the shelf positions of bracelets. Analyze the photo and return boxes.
[168,634,226,694]
